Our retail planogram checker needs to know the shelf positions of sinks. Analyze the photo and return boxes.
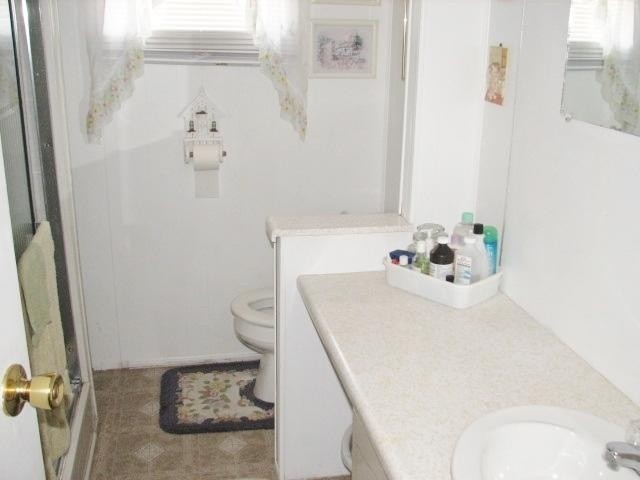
[450,402,640,480]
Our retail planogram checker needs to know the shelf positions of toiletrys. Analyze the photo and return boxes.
[389,212,502,286]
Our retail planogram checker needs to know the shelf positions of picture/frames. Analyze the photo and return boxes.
[307,19,377,79]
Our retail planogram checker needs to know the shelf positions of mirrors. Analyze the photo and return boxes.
[561,0,640,137]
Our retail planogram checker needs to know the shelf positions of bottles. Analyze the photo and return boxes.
[392,212,498,285]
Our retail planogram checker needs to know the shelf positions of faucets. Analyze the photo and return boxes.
[603,440,640,477]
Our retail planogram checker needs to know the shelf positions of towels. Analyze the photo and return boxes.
[17,220,73,480]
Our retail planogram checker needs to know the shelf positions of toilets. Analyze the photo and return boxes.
[229,287,276,405]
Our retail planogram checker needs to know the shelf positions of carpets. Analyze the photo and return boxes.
[159,359,273,434]
